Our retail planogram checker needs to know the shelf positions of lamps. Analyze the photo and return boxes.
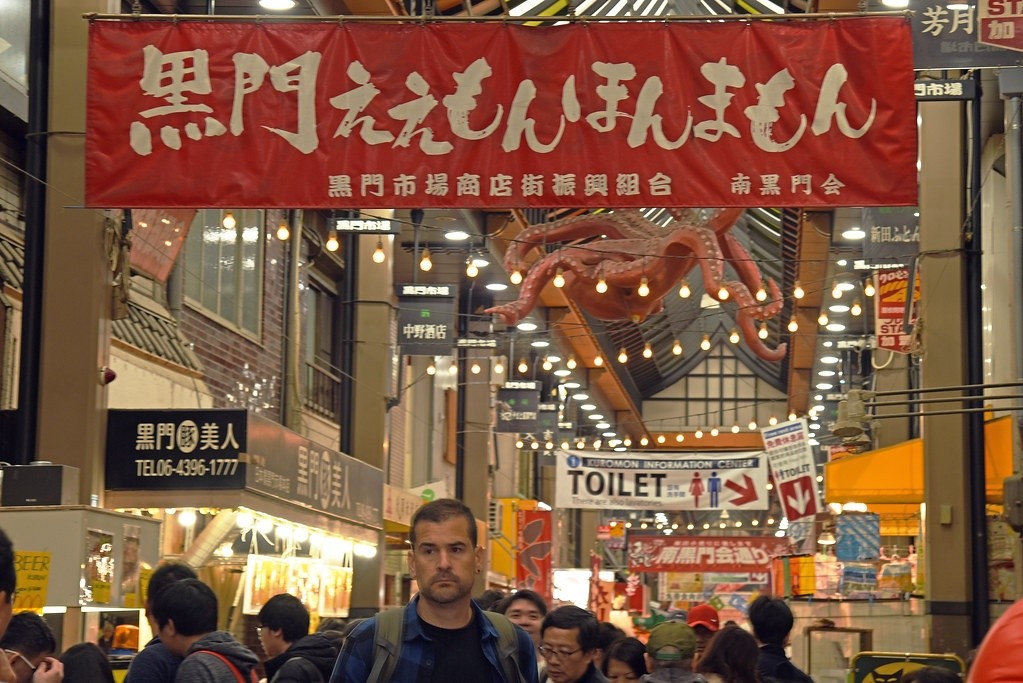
[817,521,837,546]
[832,382,1023,446]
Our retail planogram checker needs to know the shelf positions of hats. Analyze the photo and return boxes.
[646,621,695,661]
[666,610,688,621]
[687,605,720,632]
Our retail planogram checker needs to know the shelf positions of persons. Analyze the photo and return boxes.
[966,599,1023,683]
[255,594,364,683]
[121,563,267,683]
[880,547,887,559]
[331,498,538,683]
[60,642,115,683]
[99,624,114,654]
[473,589,815,683]
[907,545,917,583]
[0,527,64,683]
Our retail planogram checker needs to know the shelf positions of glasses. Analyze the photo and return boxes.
[255,626,266,636]
[537,643,581,659]
